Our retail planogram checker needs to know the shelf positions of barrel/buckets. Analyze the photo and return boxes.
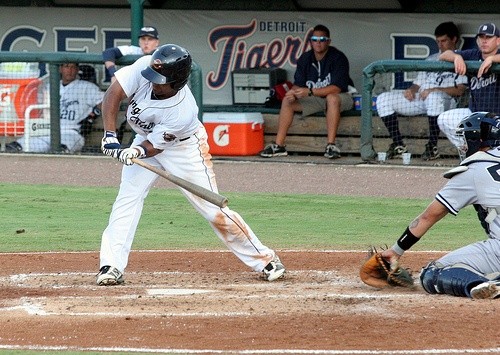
[0,72,40,135]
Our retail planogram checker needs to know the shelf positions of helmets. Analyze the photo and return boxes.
[456,112,500,163]
[141,44,194,91]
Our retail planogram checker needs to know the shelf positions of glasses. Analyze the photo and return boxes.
[310,35,329,42]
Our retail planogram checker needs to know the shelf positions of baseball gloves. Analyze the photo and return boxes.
[359,251,414,289]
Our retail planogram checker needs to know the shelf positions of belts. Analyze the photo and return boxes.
[180,137,190,141]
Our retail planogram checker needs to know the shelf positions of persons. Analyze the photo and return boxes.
[261,24,354,159]
[377,21,468,160]
[102,25,161,84]
[97,44,285,286]
[360,111,500,300]
[437,23,500,149]
[8,62,104,153]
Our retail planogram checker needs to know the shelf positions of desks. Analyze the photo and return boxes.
[82,97,134,153]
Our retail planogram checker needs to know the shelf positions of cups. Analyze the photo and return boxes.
[402,153,411,165]
[377,152,386,162]
[352,95,361,110]
[372,96,377,111]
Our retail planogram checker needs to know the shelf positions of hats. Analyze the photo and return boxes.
[475,23,500,39]
[138,26,160,38]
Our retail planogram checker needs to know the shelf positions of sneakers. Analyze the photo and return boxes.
[260,142,287,157]
[261,254,286,281]
[94,264,124,286]
[323,143,341,159]
[424,144,440,159]
[386,141,408,159]
[469,281,500,301]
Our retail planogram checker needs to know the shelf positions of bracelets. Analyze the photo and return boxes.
[393,226,420,255]
[308,88,312,96]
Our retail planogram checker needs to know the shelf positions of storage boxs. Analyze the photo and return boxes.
[202,112,265,156]
[0,71,41,136]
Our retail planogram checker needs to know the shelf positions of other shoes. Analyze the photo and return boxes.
[6,141,22,153]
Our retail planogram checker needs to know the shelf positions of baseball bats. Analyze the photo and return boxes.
[130,159,229,209]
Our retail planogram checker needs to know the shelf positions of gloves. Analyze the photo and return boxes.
[77,115,96,136]
[101,130,122,157]
[119,145,148,165]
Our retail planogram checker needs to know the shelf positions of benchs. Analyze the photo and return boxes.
[201,103,459,156]
